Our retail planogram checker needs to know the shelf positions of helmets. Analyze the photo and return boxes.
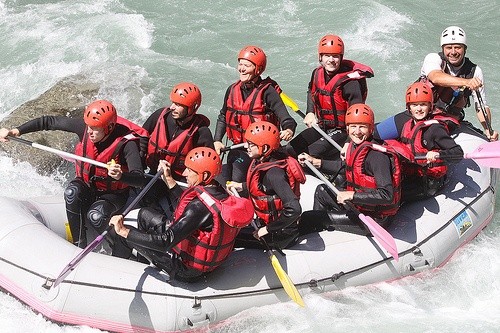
[440,26,468,50]
[238,46,267,77]
[317,35,345,62]
[344,104,374,130]
[170,82,202,116]
[83,100,117,134]
[406,81,434,113]
[184,147,222,184]
[244,122,280,157]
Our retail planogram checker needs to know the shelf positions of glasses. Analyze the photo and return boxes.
[247,143,255,149]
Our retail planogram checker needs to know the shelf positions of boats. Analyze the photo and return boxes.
[0,132,497,333]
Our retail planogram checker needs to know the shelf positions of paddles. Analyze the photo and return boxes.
[299,153,399,265]
[143,171,188,187]
[52,167,164,288]
[414,141,500,168]
[265,75,346,156]
[225,181,306,308]
[219,131,287,152]
[4,134,115,172]
[469,85,494,136]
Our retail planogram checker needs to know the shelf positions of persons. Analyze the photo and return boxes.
[109,147,255,283]
[213,45,297,198]
[372,26,499,142]
[234,120,301,249]
[287,34,363,178]
[0,101,144,259]
[298,102,402,236]
[142,80,217,184]
[400,81,463,201]
